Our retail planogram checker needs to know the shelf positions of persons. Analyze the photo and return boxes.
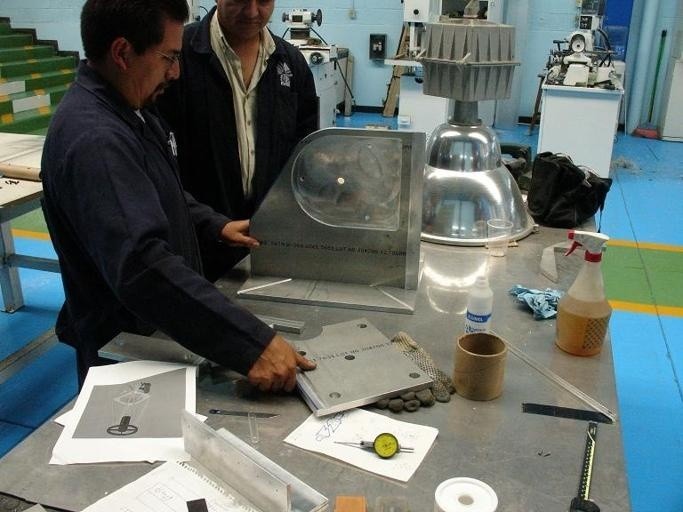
[37,1,316,399]
[145,0,319,283]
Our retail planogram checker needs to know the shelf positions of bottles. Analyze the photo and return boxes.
[462,275,493,336]
[248,411,260,444]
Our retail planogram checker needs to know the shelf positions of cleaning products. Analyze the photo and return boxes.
[555,229,611,355]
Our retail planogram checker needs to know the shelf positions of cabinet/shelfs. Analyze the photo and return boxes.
[302,47,350,128]
[539,75,624,178]
[659,57,683,143]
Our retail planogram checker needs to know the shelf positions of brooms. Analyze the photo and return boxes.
[632,30,667,141]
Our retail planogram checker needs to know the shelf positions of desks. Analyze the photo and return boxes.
[0,130,631,512]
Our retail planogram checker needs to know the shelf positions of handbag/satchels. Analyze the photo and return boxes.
[527,152,612,228]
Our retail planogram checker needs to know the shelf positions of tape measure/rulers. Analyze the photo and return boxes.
[570,422,600,512]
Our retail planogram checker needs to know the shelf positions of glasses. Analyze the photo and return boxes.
[153,47,179,65]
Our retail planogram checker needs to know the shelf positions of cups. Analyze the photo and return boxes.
[487,219,514,257]
